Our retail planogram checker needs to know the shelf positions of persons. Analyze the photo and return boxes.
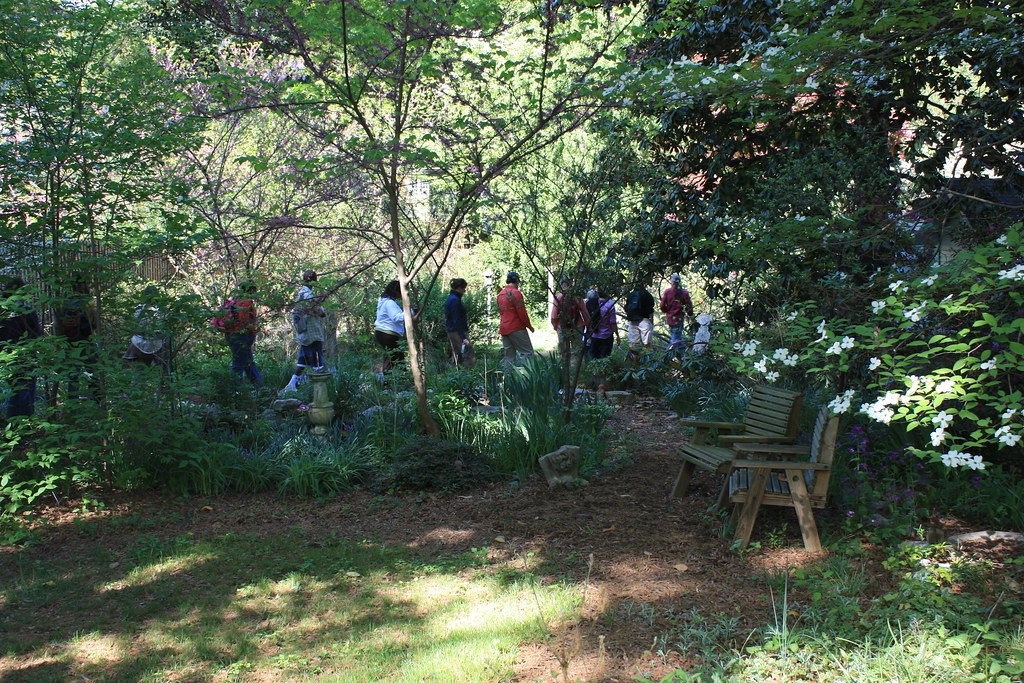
[624,282,655,362]
[284,270,326,392]
[51,276,107,405]
[660,274,696,360]
[121,285,173,383]
[213,280,263,399]
[551,279,622,361]
[374,281,419,388]
[0,276,43,418]
[497,273,534,382]
[445,279,475,369]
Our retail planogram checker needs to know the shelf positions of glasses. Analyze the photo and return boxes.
[461,287,467,295]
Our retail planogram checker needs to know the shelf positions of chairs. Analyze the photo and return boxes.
[670,383,804,514]
[726,403,840,552]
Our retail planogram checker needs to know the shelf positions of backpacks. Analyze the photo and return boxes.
[134,301,166,338]
[291,290,312,334]
[666,288,684,327]
[585,290,603,334]
[211,295,245,334]
[557,291,581,329]
[623,289,648,323]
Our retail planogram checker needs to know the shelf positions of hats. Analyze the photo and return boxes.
[671,275,681,283]
[507,272,522,279]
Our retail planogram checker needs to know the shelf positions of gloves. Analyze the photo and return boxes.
[687,313,697,324]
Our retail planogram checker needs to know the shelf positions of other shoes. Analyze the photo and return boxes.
[672,357,680,364]
[284,385,300,392]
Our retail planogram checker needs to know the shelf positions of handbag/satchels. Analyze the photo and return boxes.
[461,342,479,369]
